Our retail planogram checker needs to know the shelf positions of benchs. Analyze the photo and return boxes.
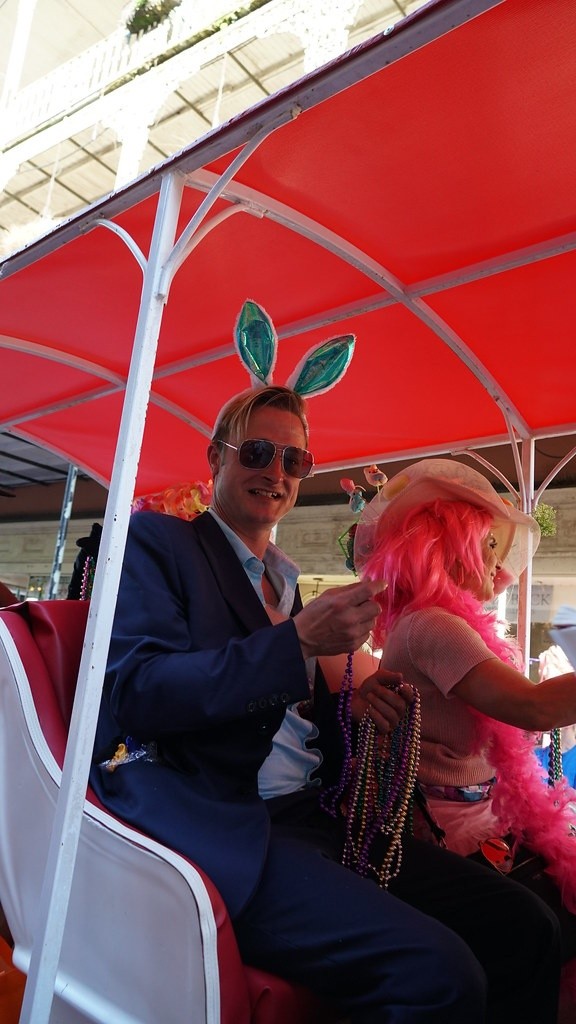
[0,600,322,1024]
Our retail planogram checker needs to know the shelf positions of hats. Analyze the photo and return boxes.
[354,458,542,594]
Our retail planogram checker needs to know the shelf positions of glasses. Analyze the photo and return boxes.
[216,438,315,479]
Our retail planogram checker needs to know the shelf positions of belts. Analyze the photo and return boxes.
[415,778,497,802]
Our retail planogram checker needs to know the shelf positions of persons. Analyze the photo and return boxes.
[354,460,576,1023]
[90,386,563,1023]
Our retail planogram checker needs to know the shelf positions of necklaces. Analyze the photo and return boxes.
[548,728,562,787]
[79,556,94,600]
[323,651,421,891]
